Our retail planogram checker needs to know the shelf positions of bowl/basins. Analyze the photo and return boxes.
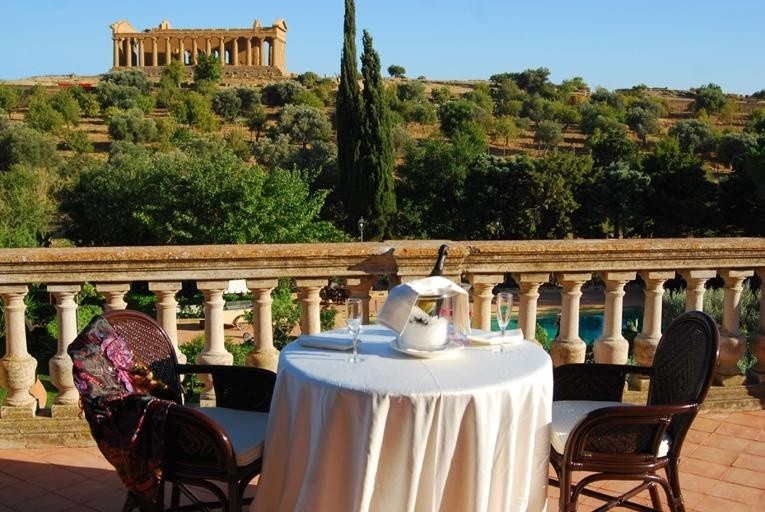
[397,298,450,351]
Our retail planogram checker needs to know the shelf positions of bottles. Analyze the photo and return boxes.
[416,244,448,325]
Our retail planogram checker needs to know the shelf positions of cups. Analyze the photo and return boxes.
[495,293,513,353]
[347,298,364,363]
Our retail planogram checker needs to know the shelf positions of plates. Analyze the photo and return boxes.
[391,340,465,359]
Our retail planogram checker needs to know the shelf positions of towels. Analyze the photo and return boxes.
[378,276,471,337]
[467,329,523,344]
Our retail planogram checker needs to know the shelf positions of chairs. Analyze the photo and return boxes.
[550,310,715,512]
[75,309,277,511]
